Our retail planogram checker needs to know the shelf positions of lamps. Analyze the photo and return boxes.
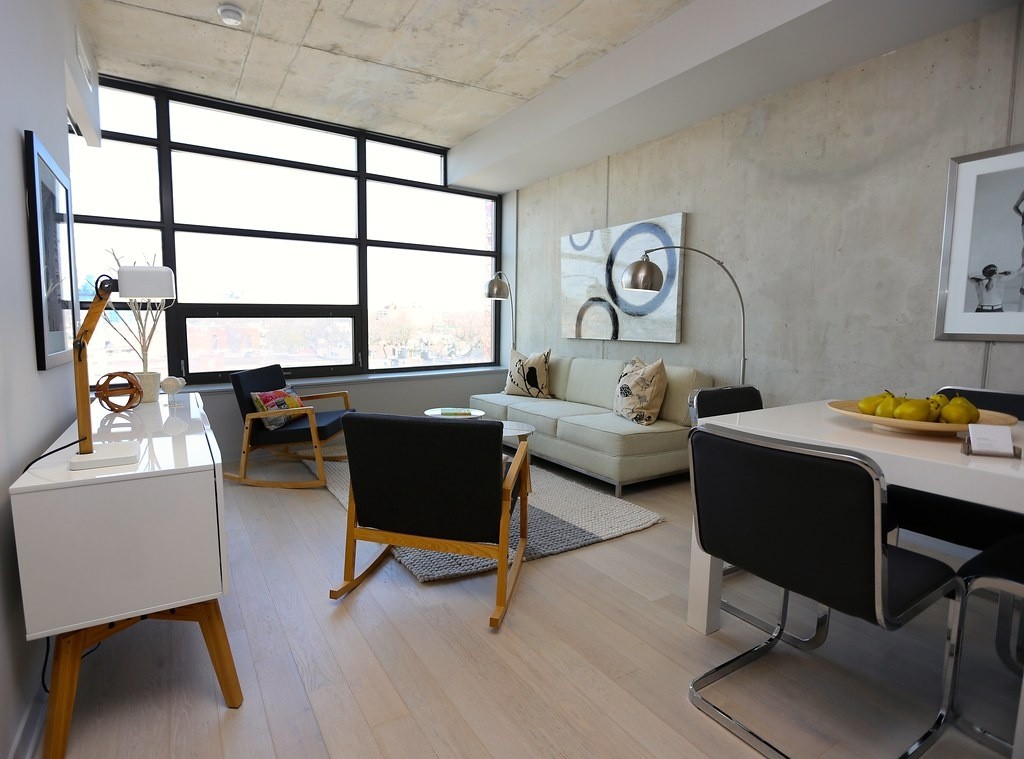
[620,245,746,385]
[217,4,245,26]
[67,265,176,470]
[486,271,514,349]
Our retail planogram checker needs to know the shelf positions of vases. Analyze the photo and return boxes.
[129,372,160,403]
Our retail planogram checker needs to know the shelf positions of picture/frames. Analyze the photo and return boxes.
[25,130,82,371]
[933,144,1024,345]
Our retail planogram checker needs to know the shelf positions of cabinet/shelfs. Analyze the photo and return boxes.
[9,392,244,759]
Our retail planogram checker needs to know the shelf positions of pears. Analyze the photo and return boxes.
[858,390,980,423]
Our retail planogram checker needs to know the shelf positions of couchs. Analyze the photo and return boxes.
[470,354,715,497]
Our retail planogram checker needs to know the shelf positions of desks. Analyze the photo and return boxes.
[687,400,1023,636]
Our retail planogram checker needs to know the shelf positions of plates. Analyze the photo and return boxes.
[423,407,485,420]
[827,399,1018,436]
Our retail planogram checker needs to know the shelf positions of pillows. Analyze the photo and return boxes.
[613,356,668,427]
[249,384,306,431]
[500,347,553,399]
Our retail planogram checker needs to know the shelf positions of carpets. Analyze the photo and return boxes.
[295,445,665,584]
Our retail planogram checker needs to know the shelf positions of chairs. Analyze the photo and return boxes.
[686,385,1024,759]
[329,413,530,628]
[224,364,350,489]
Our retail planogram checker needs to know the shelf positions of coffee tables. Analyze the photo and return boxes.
[425,408,484,420]
[501,420,536,494]
